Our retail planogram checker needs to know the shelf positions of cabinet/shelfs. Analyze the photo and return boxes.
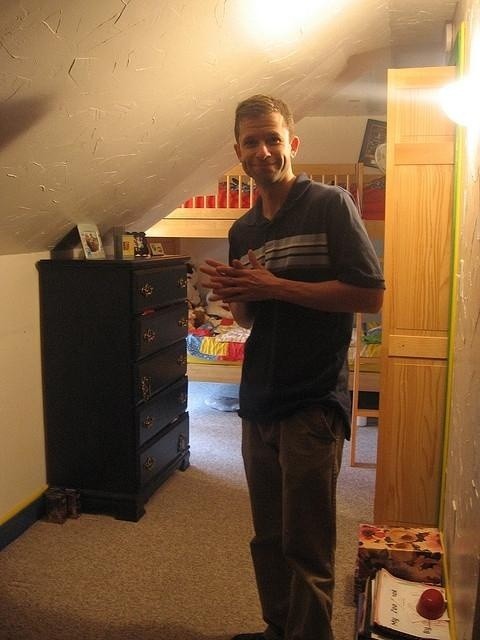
[38,254,192,524]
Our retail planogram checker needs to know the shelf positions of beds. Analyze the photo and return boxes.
[145,163,385,394]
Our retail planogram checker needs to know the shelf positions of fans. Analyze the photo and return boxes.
[375,142,386,175]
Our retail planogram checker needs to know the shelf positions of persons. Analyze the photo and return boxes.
[196,92,389,640]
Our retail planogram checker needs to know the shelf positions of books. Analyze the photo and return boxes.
[357,567,452,640]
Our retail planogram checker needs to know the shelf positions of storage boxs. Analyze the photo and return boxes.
[353,558,442,605]
[357,522,444,584]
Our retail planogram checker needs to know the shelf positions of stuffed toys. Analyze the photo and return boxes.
[185,262,235,337]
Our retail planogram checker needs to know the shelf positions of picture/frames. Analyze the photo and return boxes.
[358,118,386,169]
[76,224,106,260]
[150,242,165,256]
